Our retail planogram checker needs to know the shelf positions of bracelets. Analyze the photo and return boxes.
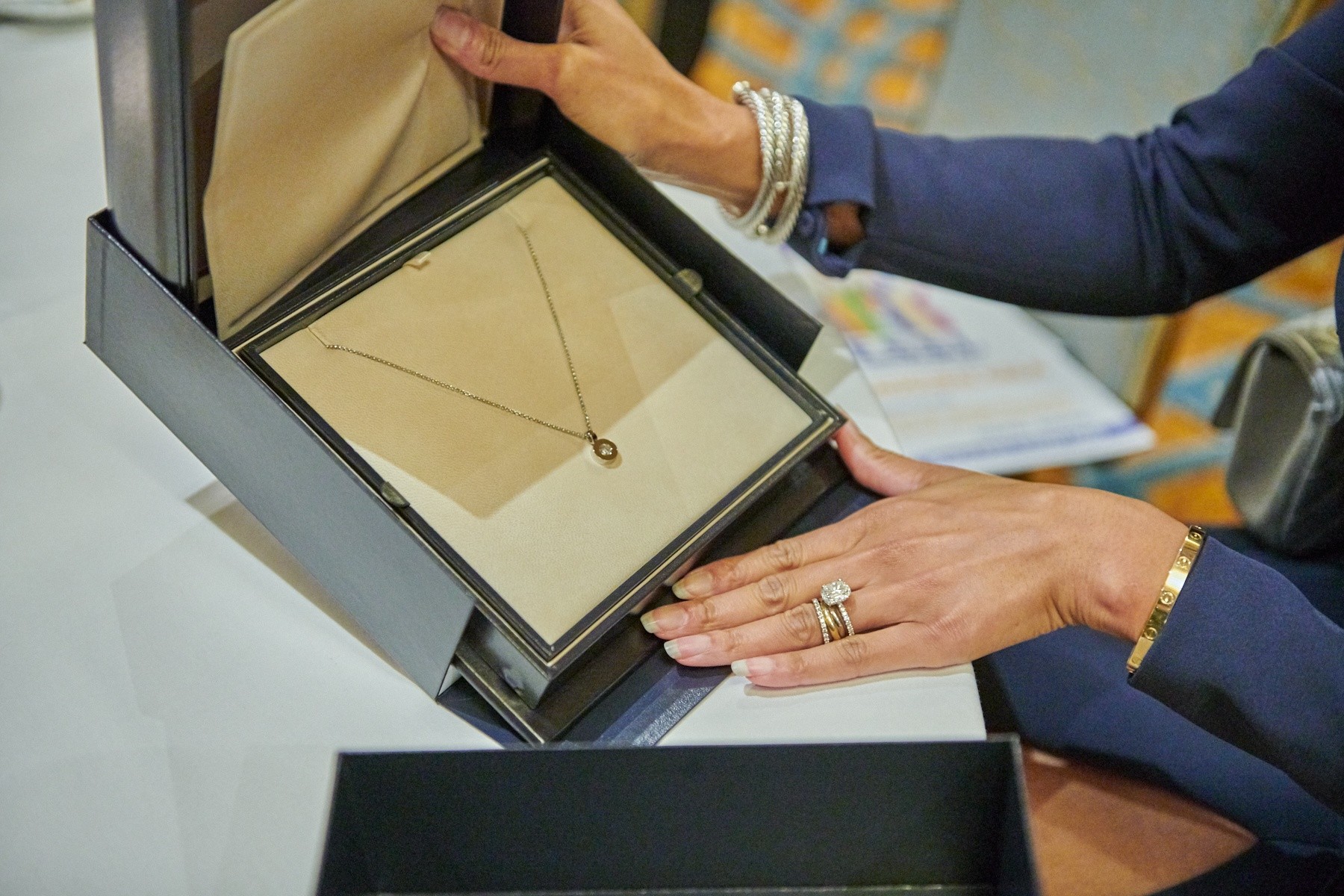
[716,80,811,246]
[1126,524,1206,673]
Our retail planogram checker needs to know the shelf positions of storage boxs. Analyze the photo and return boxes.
[83,0,874,752]
[314,737,1047,896]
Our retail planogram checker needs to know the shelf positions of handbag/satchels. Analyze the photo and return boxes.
[1211,324,1344,559]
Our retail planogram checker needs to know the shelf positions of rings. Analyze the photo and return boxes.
[811,578,855,644]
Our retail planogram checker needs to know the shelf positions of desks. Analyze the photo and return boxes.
[1,18,1016,896]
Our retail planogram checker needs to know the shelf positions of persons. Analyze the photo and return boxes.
[427,0,1344,896]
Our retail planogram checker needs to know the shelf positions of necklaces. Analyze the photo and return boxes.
[325,225,621,464]
